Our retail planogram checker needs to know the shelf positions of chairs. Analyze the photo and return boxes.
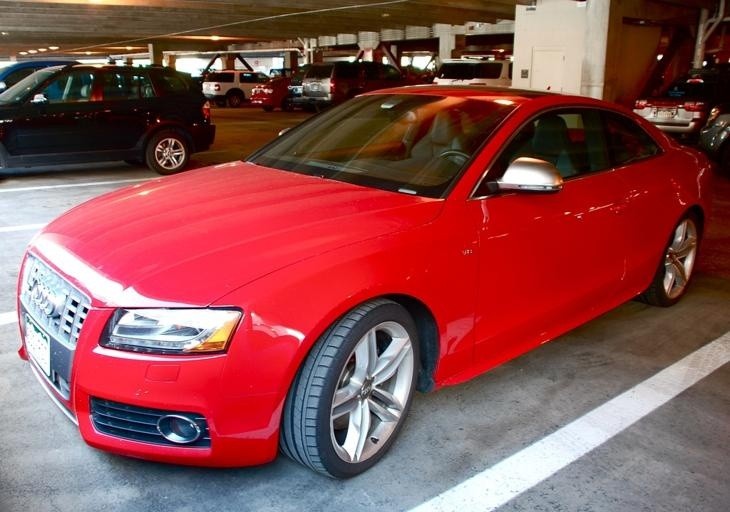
[408,108,471,164]
[520,115,588,177]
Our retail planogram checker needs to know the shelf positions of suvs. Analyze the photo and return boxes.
[0,65,218,179]
[202,66,270,109]
[431,56,513,87]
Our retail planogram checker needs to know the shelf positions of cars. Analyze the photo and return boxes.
[630,69,711,136]
[0,57,82,94]
[250,67,294,114]
[289,76,304,105]
[699,102,730,171]
[11,74,718,485]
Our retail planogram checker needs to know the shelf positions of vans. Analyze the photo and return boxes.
[301,63,412,103]
